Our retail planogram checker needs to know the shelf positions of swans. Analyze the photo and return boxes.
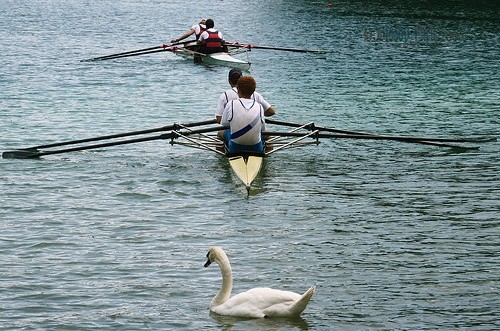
[204,246,316,319]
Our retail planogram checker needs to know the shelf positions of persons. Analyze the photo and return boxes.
[197,18,226,54]
[216,67,275,124]
[217,75,265,155]
[170,19,207,45]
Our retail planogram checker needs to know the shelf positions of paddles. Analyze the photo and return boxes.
[2,119,500,161]
[80,39,327,63]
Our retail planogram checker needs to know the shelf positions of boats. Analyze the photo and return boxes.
[226,155,264,195]
[195,39,253,71]
